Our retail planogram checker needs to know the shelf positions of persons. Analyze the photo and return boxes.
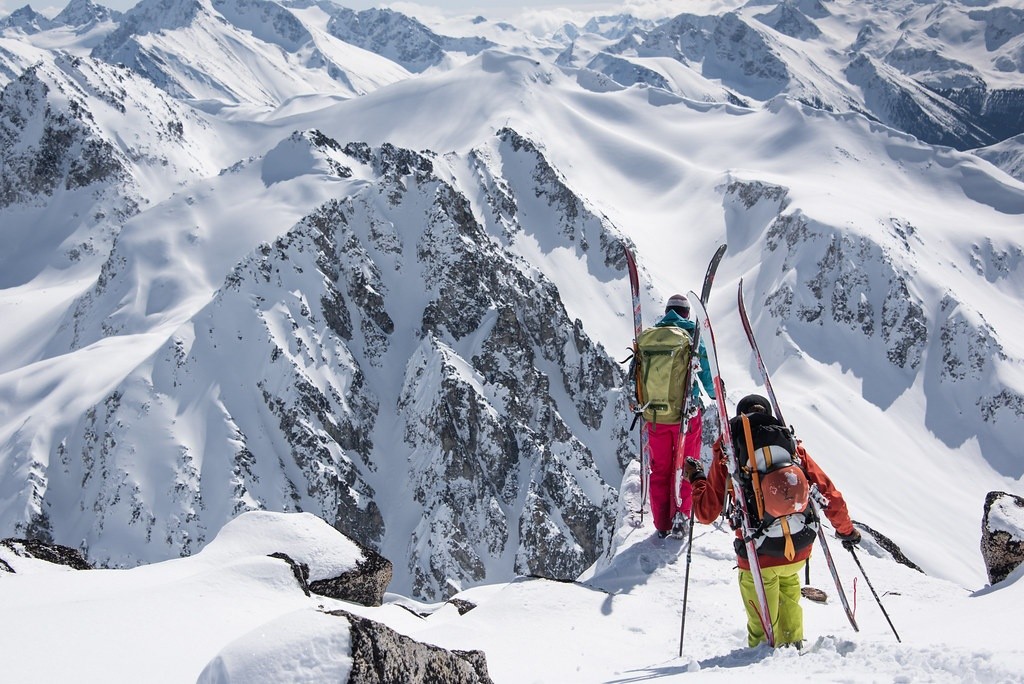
[682,395,861,650]
[631,295,718,539]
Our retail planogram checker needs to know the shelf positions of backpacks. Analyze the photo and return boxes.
[636,321,700,425]
[721,415,821,559]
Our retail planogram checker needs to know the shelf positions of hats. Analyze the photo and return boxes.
[737,394,772,416]
[666,295,690,313]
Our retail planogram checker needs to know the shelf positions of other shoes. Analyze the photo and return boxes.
[658,529,670,539]
[672,510,688,539]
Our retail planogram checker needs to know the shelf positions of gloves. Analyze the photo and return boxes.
[683,456,706,484]
[835,528,861,551]
